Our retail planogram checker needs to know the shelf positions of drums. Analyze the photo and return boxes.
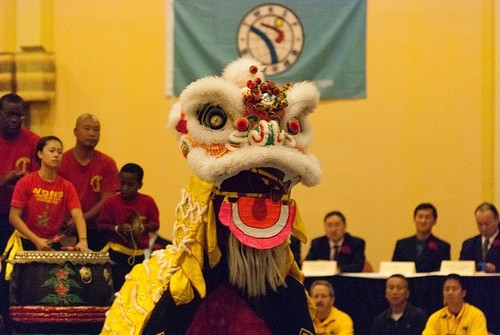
[9,250,115,311]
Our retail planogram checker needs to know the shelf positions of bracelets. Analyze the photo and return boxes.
[79,238,87,239]
[115,225,120,235]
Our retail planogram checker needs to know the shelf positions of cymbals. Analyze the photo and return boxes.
[127,209,146,245]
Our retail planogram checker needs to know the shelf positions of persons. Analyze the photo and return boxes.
[304,202,500,335]
[0,93,173,308]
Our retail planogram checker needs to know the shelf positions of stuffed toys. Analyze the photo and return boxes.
[98,52,315,335]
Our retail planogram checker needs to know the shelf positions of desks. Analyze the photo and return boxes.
[305,272,500,335]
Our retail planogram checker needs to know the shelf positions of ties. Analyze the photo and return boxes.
[483,240,490,259]
[333,246,339,260]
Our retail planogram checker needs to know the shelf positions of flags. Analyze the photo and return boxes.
[166,0,367,99]
